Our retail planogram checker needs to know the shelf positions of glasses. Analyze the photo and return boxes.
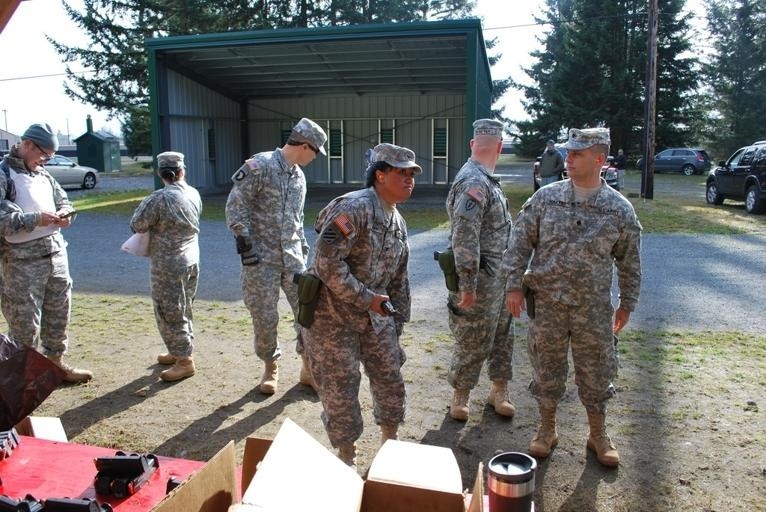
[30,141,54,162]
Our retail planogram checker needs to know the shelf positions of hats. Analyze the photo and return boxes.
[156,151,185,168]
[293,118,329,158]
[20,124,60,152]
[558,127,612,150]
[472,118,504,140]
[372,143,423,176]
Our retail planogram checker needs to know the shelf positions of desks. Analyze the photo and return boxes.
[2,435,535,511]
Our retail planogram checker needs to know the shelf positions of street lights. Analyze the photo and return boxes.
[2,109,7,131]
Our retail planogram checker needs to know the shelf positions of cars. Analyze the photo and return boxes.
[36,155,101,189]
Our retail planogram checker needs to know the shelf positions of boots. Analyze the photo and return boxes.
[586,411,620,469]
[339,441,359,471]
[47,356,93,384]
[529,406,560,459]
[488,381,515,417]
[450,387,471,422]
[259,359,279,395]
[157,353,179,366]
[380,427,399,446]
[299,355,319,395]
[160,355,196,383]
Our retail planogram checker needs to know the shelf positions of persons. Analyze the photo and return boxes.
[130,151,204,384]
[538,140,565,188]
[0,121,94,385]
[225,117,329,395]
[301,141,423,470]
[444,117,517,423]
[500,127,644,467]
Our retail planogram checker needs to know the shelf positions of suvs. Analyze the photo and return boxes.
[704,141,765,215]
[635,148,710,176]
[531,143,621,196]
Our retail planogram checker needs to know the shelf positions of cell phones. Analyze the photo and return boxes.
[61,210,77,219]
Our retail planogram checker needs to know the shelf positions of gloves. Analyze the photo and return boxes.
[236,234,262,266]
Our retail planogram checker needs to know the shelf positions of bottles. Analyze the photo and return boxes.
[487,451,538,512]
